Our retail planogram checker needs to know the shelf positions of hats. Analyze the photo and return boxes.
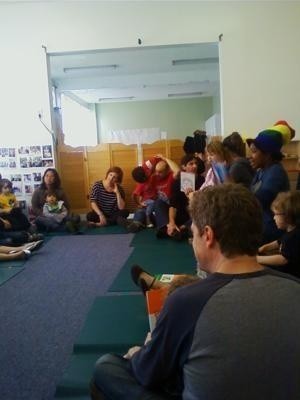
[246,130,285,158]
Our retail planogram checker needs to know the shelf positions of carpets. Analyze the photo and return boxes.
[0,221,196,400]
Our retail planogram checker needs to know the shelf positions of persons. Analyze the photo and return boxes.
[144,275,202,345]
[130,121,300,296]
[0,179,22,232]
[86,166,129,228]
[89,181,300,400]
[31,168,80,233]
[0,173,44,244]
[42,189,68,224]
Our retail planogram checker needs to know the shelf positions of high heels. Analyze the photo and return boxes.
[10,233,43,257]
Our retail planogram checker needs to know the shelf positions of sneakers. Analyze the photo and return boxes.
[131,264,156,296]
[46,221,168,239]
